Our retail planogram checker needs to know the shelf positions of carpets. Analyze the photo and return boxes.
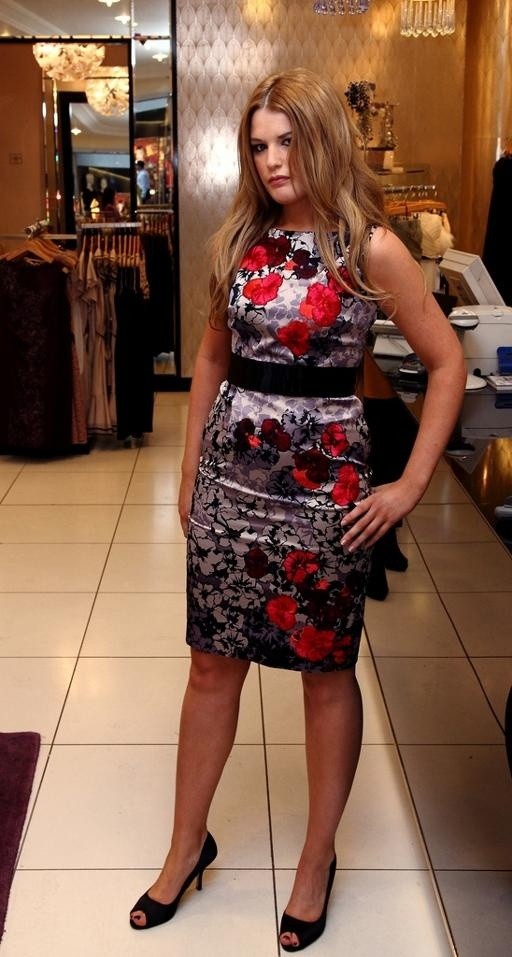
[0,731,43,945]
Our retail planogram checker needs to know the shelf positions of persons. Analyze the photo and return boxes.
[101,178,113,206]
[136,159,151,203]
[83,172,98,211]
[131,67,469,952]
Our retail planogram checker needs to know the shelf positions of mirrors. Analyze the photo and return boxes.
[0,0,180,378]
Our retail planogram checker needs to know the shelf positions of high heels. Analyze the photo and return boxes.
[280,855,336,953]
[131,831,217,930]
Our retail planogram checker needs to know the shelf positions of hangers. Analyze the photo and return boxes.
[0,207,174,295]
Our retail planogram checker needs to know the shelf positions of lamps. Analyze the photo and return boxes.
[32,42,130,119]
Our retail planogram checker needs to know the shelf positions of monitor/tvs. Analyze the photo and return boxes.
[438,249,506,306]
